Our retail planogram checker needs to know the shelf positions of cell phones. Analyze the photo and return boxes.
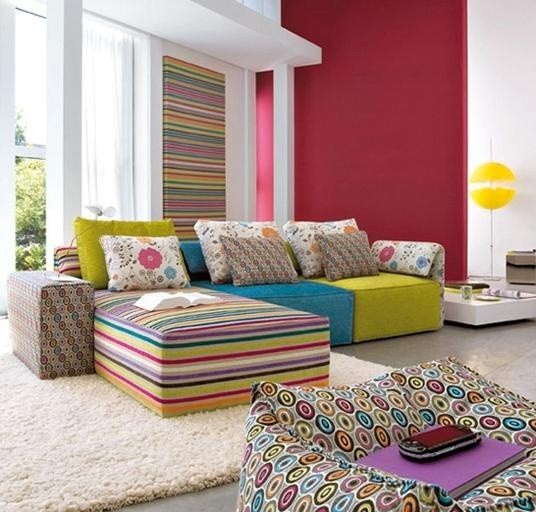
[399,423,482,462]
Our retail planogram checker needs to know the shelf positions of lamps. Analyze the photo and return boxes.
[467,160,516,280]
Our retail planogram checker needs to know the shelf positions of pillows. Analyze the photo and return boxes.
[218,229,379,286]
[98,234,191,292]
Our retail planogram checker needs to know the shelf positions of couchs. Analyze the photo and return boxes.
[4,215,446,421]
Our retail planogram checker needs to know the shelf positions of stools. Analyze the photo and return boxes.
[236,352,536,511]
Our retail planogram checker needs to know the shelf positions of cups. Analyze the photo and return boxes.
[460,286,473,300]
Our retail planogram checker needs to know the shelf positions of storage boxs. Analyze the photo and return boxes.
[505,250,536,284]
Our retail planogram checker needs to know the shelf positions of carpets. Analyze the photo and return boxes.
[3,338,400,511]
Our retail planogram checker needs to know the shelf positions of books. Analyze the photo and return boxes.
[131,291,224,312]
[352,427,527,500]
[482,287,536,299]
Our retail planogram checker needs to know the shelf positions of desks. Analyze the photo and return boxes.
[441,286,535,328]
